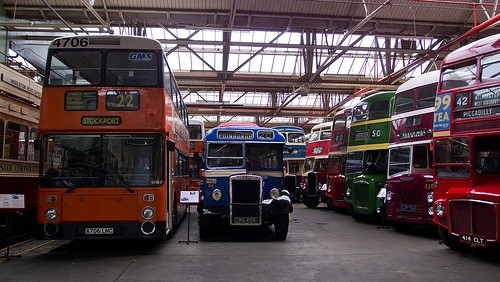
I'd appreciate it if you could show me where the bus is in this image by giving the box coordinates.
[272,33,500,251]
[0,35,206,241]
[198,126,293,242]
